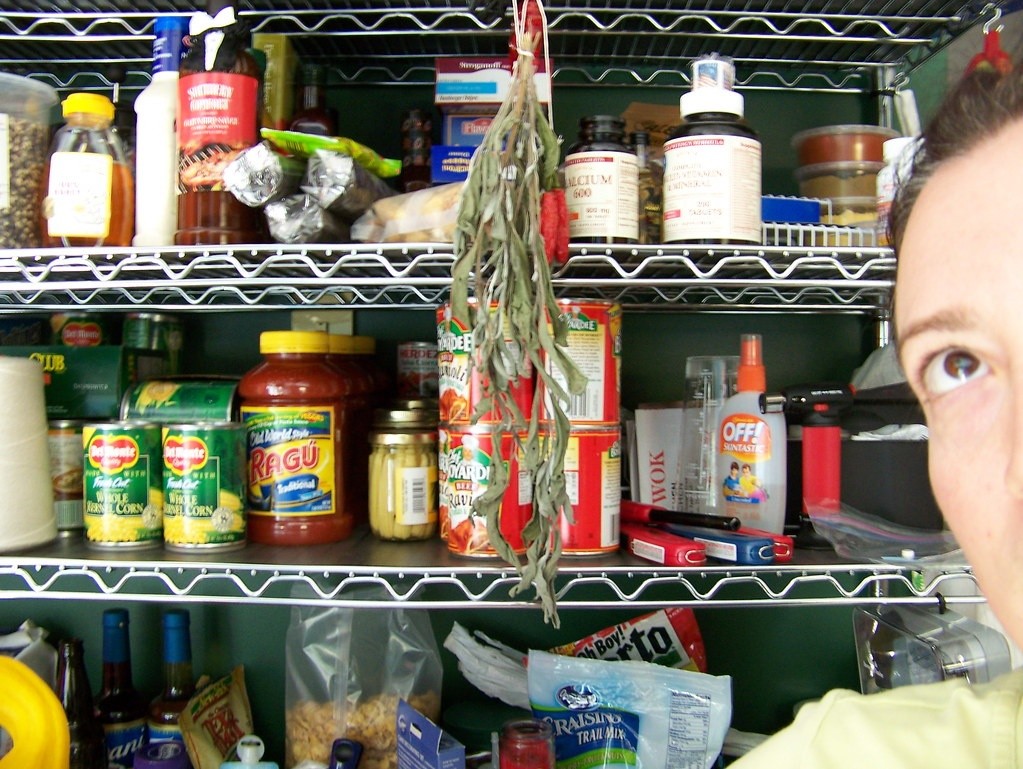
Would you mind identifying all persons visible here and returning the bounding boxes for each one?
[716,63,1023,769]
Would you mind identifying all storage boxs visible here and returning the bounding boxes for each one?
[253,33,299,133]
[431,57,554,184]
[1,343,179,419]
[763,197,821,224]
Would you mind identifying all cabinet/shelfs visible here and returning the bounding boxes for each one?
[0,0,1019,610]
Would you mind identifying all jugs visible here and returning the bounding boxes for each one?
[679,353,739,517]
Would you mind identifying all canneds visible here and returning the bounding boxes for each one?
[30,299,623,564]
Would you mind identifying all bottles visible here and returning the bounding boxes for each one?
[562,53,762,265]
[714,333,789,542]
[0,17,334,249]
[91,608,147,769]
[142,609,198,760]
[56,639,109,769]
